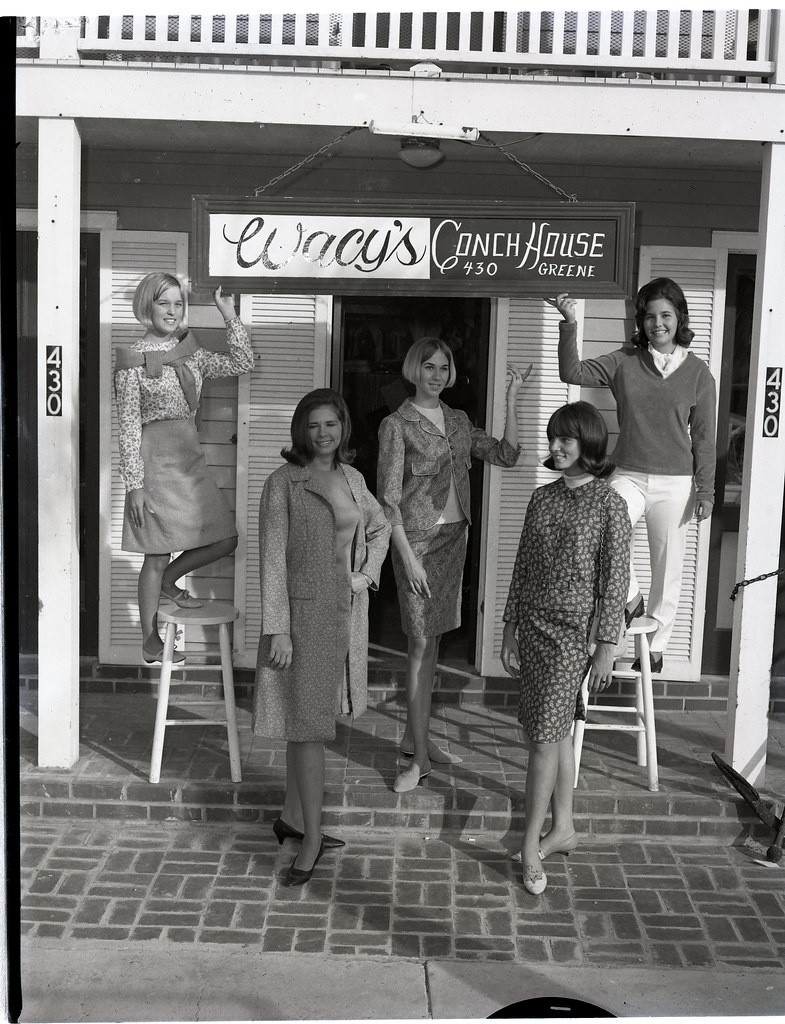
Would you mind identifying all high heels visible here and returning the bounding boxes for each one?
[287,839,325,886]
[273,818,345,848]
[522,865,547,895]
[511,831,577,862]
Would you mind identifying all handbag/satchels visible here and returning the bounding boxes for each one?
[587,596,627,657]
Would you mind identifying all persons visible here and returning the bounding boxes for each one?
[498,401,633,895]
[376,336,532,793]
[544,275,716,673]
[112,272,255,663]
[251,387,393,888]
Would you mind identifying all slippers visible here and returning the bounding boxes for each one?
[400,747,463,764]
[393,770,431,793]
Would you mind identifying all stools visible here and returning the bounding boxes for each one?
[149,602,242,784]
[571,615,659,792]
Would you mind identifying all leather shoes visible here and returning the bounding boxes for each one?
[631,653,662,674]
[624,597,644,630]
[143,644,186,663]
[160,588,204,608]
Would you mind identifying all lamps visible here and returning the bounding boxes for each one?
[400,135,446,170]
